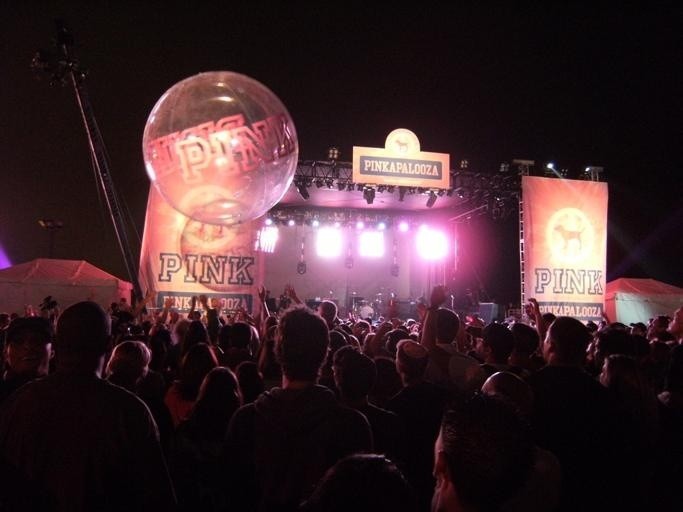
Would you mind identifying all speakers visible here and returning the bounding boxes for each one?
[307,301,321,311]
[265,298,289,316]
[480,303,504,327]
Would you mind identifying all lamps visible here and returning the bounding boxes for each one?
[457,159,469,170]
[327,146,339,161]
[293,175,501,209]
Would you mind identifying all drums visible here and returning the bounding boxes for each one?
[356,298,384,320]
[350,297,364,301]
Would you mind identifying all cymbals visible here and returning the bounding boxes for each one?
[347,291,360,295]
[373,293,386,296]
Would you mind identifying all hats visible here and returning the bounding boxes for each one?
[396,339,432,359]
[630,322,647,330]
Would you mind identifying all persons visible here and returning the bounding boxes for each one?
[0,284,683,512]
